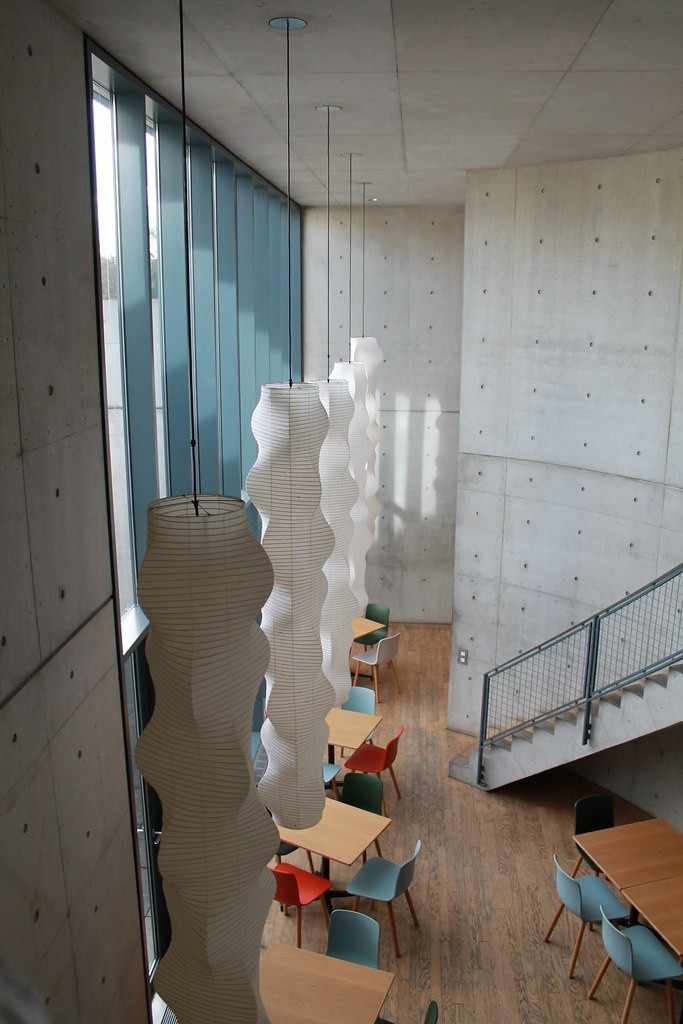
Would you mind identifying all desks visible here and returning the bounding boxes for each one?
[324,707,383,791]
[349,616,386,682]
[271,798,391,920]
[572,818,683,954]
[254,942,397,1024]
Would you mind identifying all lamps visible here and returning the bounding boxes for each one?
[133,0,384,1024]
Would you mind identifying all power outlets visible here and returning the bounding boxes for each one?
[458,647,468,665]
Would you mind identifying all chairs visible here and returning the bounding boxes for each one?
[340,773,391,864]
[345,839,422,958]
[375,1000,438,1024]
[352,632,401,703]
[341,686,383,779]
[543,854,629,978]
[344,725,404,817]
[324,908,380,972]
[354,604,397,674]
[570,793,615,882]
[266,861,334,949]
[588,905,683,1024]
